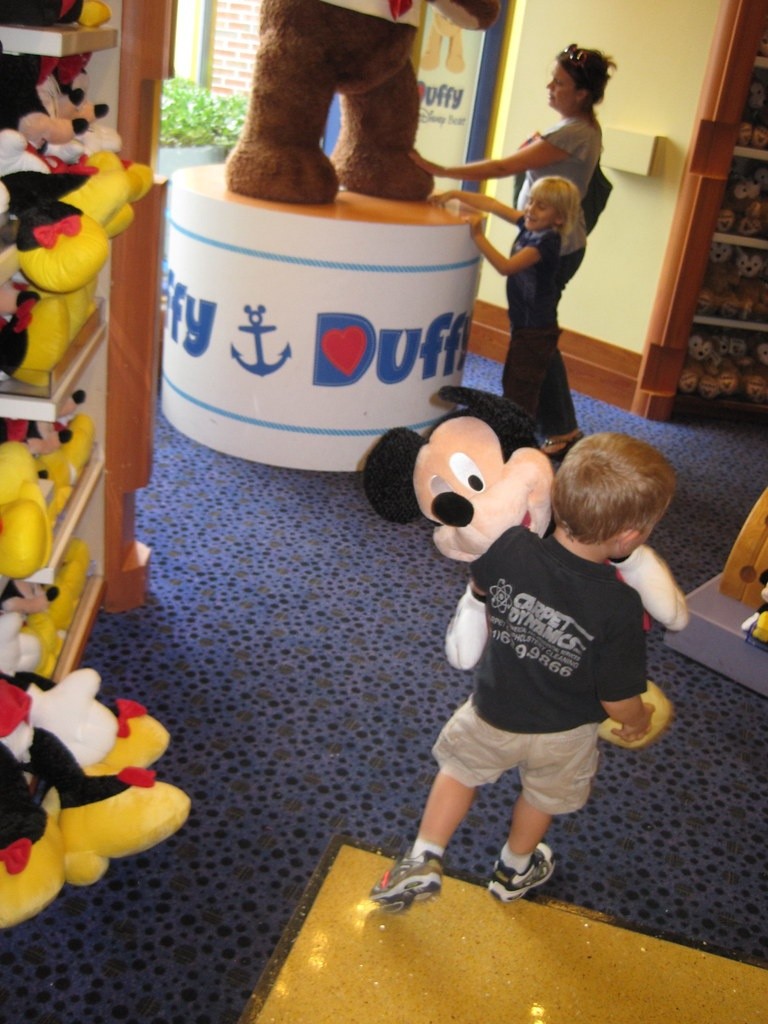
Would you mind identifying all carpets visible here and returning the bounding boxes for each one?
[236,835,768,1024]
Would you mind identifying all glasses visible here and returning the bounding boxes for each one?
[566,44,590,90]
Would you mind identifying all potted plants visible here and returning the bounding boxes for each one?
[157,76,247,174]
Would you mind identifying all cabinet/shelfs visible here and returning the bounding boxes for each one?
[673,54,768,414]
[0,22,108,685]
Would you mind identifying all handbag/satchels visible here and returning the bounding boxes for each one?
[513,147,613,238]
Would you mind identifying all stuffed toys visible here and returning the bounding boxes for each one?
[676,31,768,401]
[0,0,192,933]
[362,386,688,748]
[228,0,501,206]
[742,570,768,641]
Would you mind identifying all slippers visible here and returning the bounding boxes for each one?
[540,430,584,456]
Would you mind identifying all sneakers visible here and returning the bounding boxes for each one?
[489,843,555,903]
[369,844,443,914]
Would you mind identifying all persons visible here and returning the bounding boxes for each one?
[368,433,677,914]
[430,175,584,422]
[407,43,613,456]
